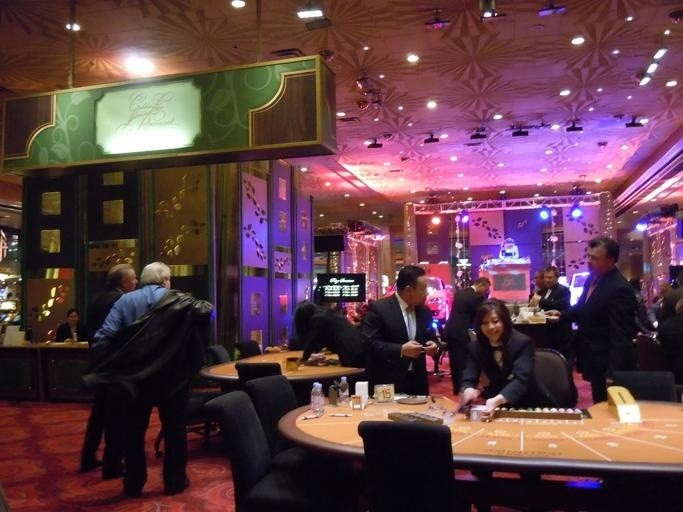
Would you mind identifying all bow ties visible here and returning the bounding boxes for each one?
[492,345,503,351]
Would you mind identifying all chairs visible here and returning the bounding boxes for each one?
[208,344,230,362]
[287,336,306,351]
[248,376,304,463]
[526,350,577,405]
[207,393,309,510]
[155,388,212,459]
[346,422,470,507]
[612,370,677,400]
[431,327,450,376]
[234,340,262,356]
[235,361,276,383]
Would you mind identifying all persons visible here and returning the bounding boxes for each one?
[293,300,366,397]
[81,263,139,480]
[458,297,559,512]
[55,308,91,342]
[445,265,683,395]
[359,265,444,395]
[544,237,640,405]
[91,261,215,497]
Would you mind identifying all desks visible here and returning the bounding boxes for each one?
[511,321,573,348]
[201,345,365,401]
[276,395,683,512]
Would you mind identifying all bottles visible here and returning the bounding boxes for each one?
[312,384,324,414]
[338,377,349,402]
[310,383,318,408]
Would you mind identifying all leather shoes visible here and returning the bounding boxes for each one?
[83,460,125,479]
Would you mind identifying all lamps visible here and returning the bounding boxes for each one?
[539,204,552,221]
[633,32,668,88]
[479,0,497,17]
[571,202,583,219]
[352,222,386,241]
[357,77,381,107]
[367,118,644,148]
[461,211,470,224]
[296,2,326,19]
[431,212,440,225]
